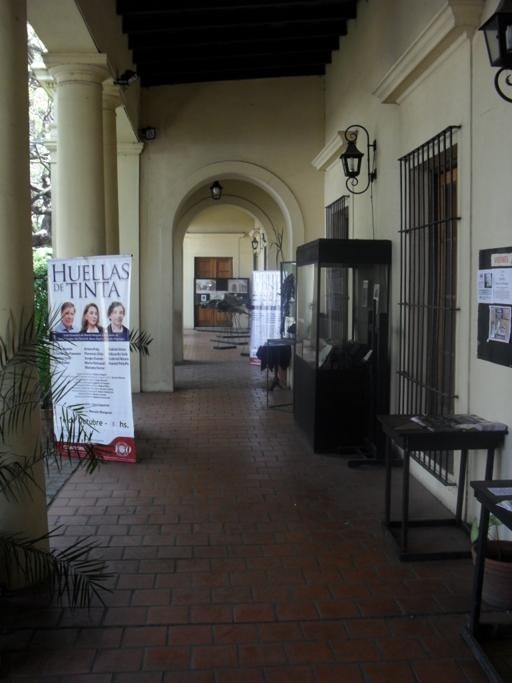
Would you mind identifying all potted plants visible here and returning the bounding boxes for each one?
[470,510,512,609]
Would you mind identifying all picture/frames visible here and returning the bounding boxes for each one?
[194,277,249,307]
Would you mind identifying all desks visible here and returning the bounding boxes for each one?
[378,414,509,562]
[255,344,291,392]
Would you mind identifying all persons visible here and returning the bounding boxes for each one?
[106,301,129,333]
[78,302,104,334]
[54,302,79,333]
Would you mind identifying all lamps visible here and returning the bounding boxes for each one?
[113,70,138,86]
[339,125,376,194]
[138,125,155,141]
[478,1,512,103]
[210,180,224,200]
[252,237,263,250]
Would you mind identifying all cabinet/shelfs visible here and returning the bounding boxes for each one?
[458,480,512,683]
